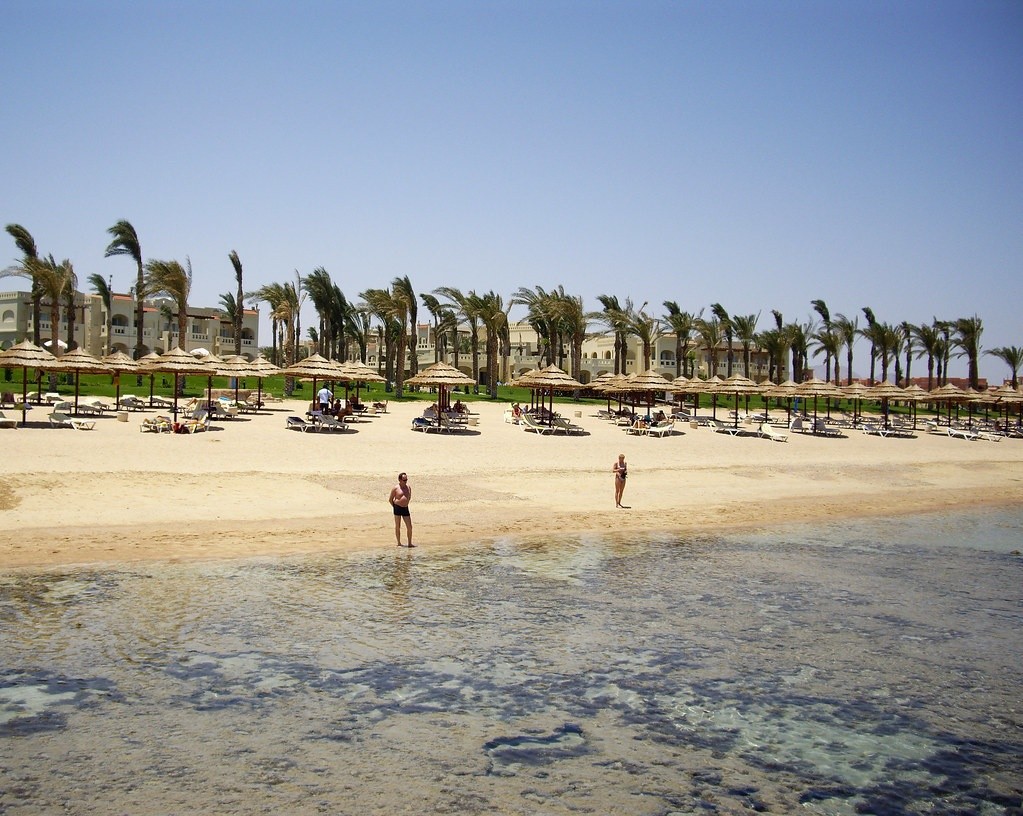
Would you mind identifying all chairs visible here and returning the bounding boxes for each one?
[0,389,264,434]
[285,399,1023,442]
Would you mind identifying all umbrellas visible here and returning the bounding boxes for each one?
[279,352,388,430]
[403,361,477,433]
[758,378,1023,434]
[671,373,767,429]
[585,369,681,426]
[513,363,587,427]
[0,339,283,427]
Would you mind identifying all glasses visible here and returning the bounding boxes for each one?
[401,479,407,482]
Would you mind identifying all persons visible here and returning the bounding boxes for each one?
[389,472,417,548]
[317,385,333,414]
[334,399,341,410]
[658,410,664,421]
[175,418,198,427]
[613,454,628,508]
[338,401,352,419]
[512,403,520,421]
[453,399,463,412]
[350,394,356,403]
[143,416,170,424]
[523,405,528,413]
